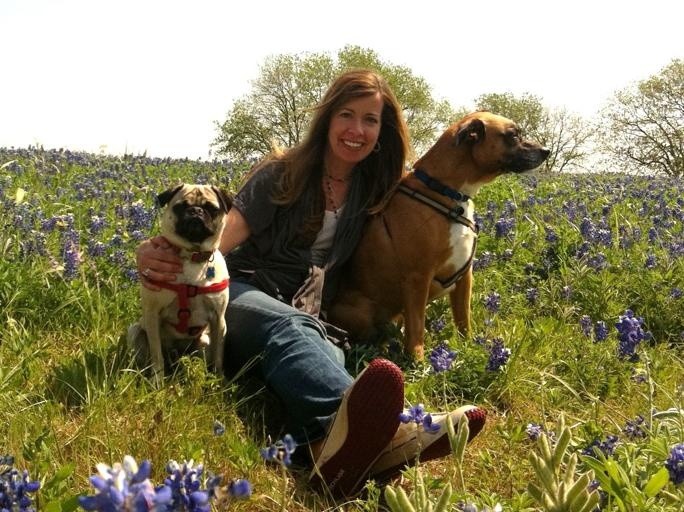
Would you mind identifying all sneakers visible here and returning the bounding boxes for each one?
[306,358,405,501]
[359,405,486,488]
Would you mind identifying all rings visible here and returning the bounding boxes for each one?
[142,268,150,277]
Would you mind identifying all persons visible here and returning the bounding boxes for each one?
[136,69,489,502]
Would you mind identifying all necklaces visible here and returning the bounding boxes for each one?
[325,177,339,220]
[327,172,351,183]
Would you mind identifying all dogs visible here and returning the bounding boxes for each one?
[124,181,235,391]
[325,109,552,370]
